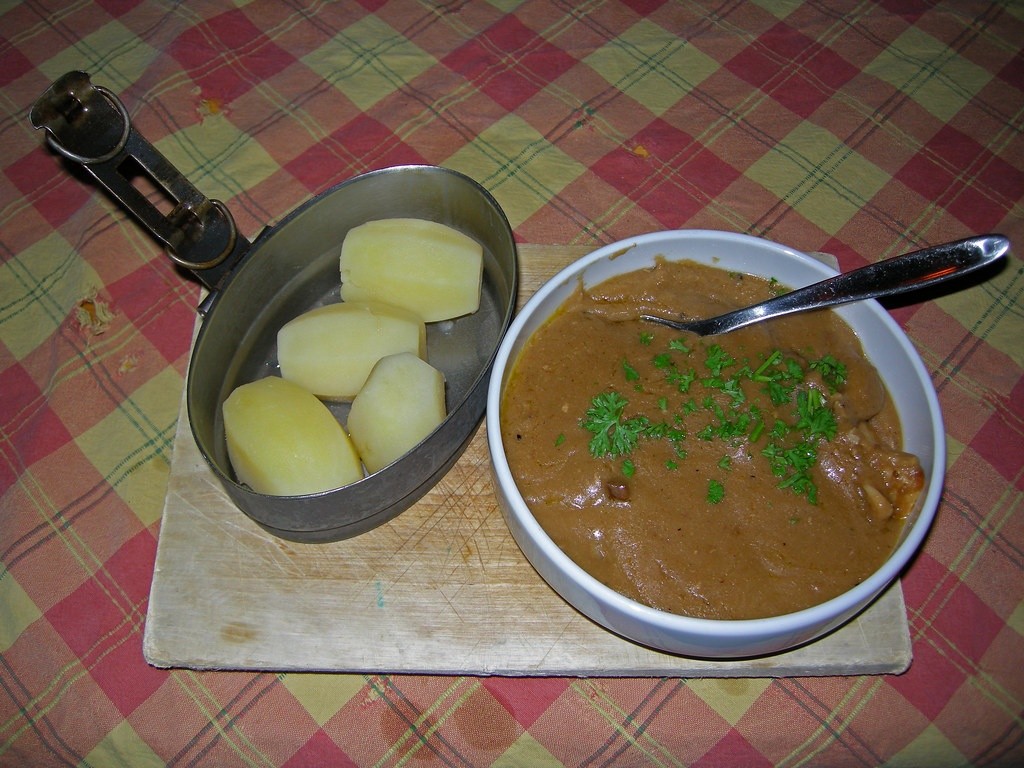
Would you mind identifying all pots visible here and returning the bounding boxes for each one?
[27,69,521,545]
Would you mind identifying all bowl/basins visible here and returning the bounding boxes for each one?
[488,229,946,656]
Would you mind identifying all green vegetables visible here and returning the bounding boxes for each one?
[579,333,848,505]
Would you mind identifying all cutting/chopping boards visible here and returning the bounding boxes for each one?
[142,240,914,676]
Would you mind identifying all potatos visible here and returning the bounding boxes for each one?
[223,217,483,496]
